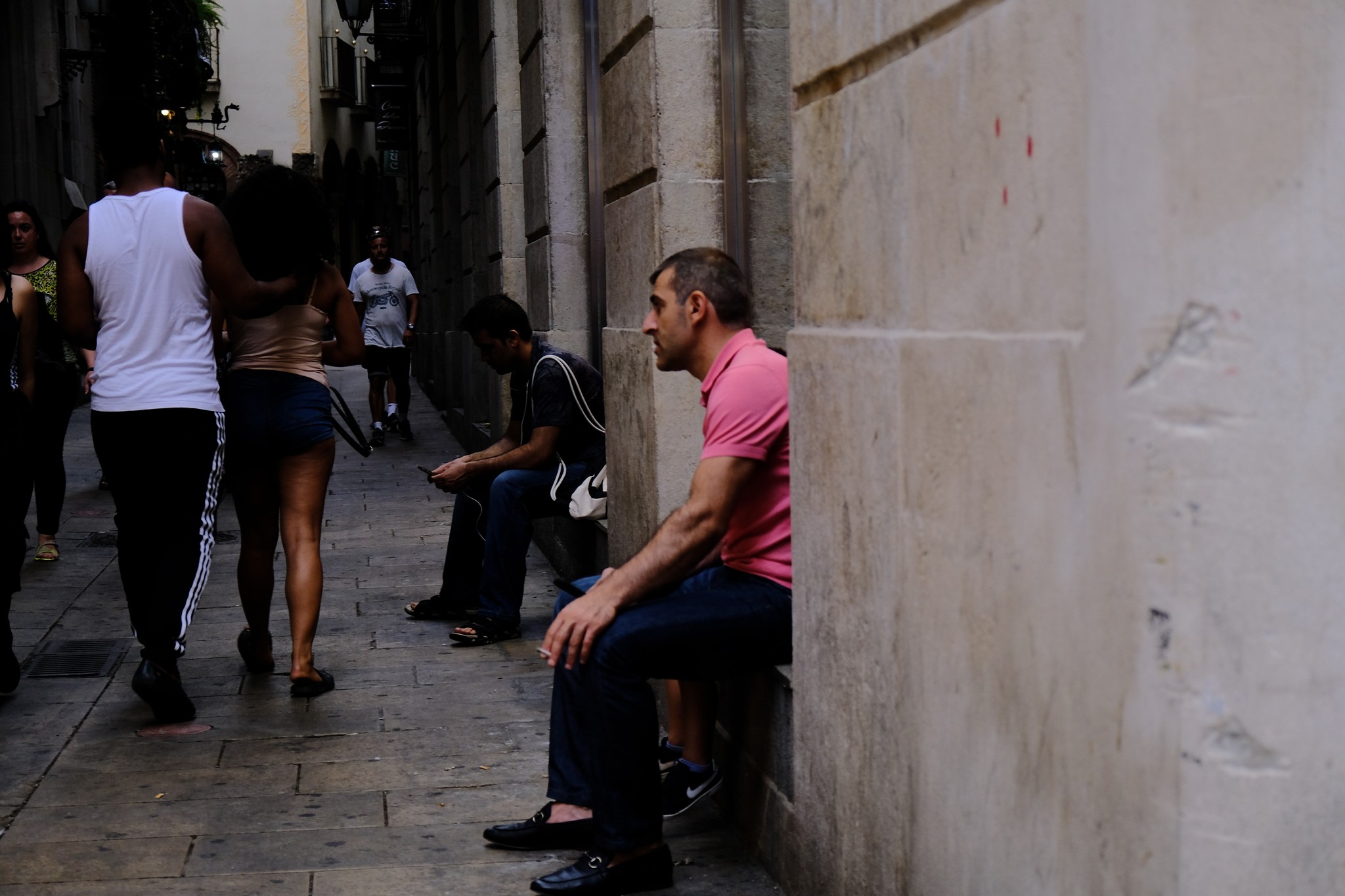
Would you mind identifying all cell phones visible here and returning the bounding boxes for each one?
[418,466,437,476]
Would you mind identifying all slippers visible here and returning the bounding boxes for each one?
[291,668,335,697]
[237,626,276,676]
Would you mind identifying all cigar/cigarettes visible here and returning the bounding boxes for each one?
[535,647,551,658]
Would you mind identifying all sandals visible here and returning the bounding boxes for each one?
[403,593,479,618]
[34,542,60,561]
[449,620,523,643]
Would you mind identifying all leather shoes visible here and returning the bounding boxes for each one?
[529,844,674,895]
[483,800,594,850]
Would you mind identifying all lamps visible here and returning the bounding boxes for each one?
[336,0,424,44]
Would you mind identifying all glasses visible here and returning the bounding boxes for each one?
[369,230,386,236]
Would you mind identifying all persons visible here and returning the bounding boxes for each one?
[653,677,721,822]
[403,294,605,644]
[482,246,787,896]
[0,97,420,722]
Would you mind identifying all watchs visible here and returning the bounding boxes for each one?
[406,324,415,331]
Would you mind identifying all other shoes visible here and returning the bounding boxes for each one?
[100,477,109,490]
[369,428,385,446]
[386,413,398,424]
[659,737,684,773]
[132,660,196,723]
[397,419,415,440]
[660,757,722,817]
